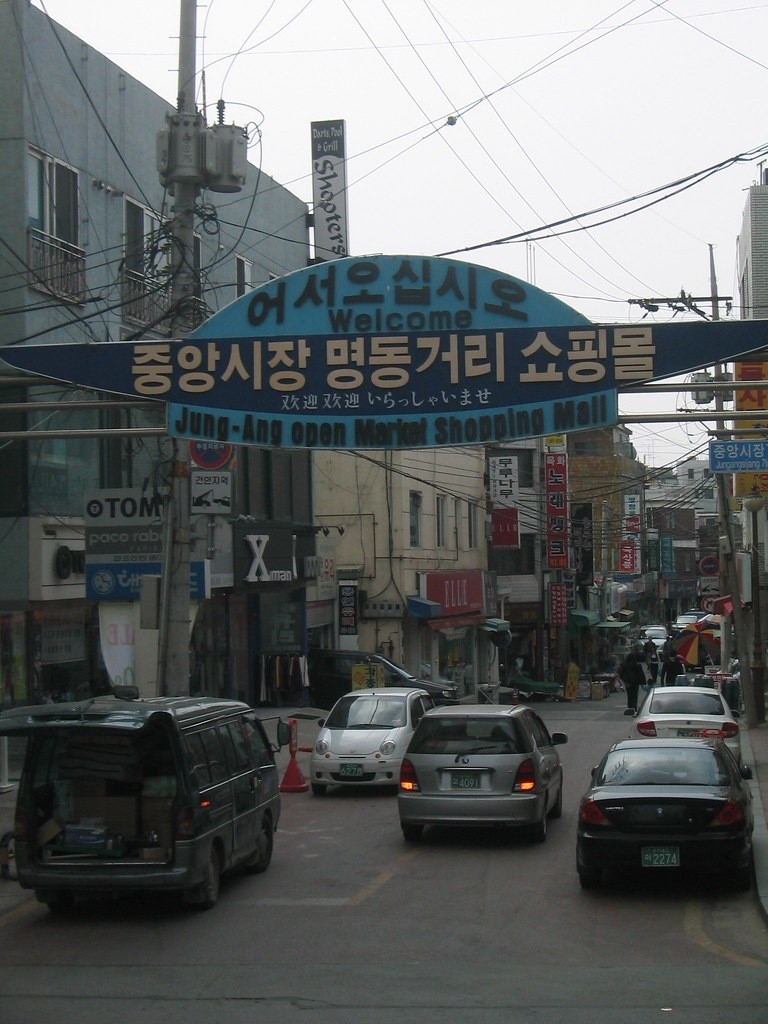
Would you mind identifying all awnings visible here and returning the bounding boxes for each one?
[567,608,635,633]
[480,618,510,632]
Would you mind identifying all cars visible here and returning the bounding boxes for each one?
[629,625,673,662]
[398,704,570,842]
[668,611,706,633]
[575,737,754,889]
[310,685,437,796]
[623,684,741,764]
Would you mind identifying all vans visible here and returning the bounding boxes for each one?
[0,684,292,916]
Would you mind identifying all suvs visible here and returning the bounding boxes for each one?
[292,649,460,708]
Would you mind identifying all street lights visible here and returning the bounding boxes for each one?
[743,482,765,720]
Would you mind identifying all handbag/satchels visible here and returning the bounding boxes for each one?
[659,665,662,676]
[636,664,646,685]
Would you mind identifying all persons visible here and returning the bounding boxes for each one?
[597,635,686,713]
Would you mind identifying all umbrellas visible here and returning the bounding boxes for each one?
[672,620,722,666]
